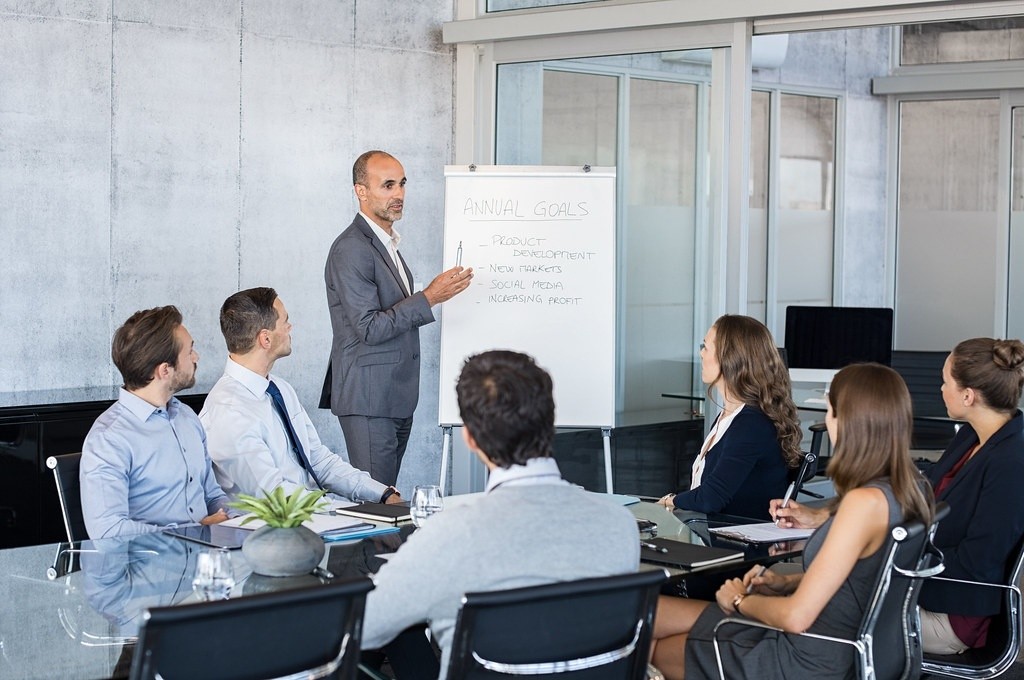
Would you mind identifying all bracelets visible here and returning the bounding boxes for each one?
[663,492,676,505]
[731,593,752,608]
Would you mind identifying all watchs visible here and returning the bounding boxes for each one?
[380,485,401,505]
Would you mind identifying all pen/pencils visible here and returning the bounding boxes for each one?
[640,541,669,553]
[747,566,766,594]
[798,489,826,498]
[456,241,463,276]
[317,566,334,578]
[774,481,795,526]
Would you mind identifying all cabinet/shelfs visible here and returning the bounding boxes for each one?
[0,383,214,550]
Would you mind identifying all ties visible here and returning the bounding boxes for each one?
[266,380,327,496]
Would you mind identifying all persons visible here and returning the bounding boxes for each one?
[765,338,1024,657]
[80,304,246,543]
[362,350,641,679]
[317,150,475,488]
[81,534,207,642]
[195,287,408,516]
[649,310,807,603]
[646,362,935,680]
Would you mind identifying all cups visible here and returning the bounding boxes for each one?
[410,485,443,529]
[191,548,235,602]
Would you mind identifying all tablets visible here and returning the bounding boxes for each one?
[161,524,257,550]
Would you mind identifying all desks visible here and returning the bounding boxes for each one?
[662,382,972,425]
[1,492,813,680]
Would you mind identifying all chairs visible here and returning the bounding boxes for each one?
[786,450,817,501]
[489,417,705,496]
[46,453,160,648]
[920,545,1024,680]
[891,351,968,450]
[358,568,670,680]
[47,543,80,580]
[713,501,952,680]
[131,576,375,680]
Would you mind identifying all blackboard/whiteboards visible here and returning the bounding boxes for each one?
[438,164,618,428]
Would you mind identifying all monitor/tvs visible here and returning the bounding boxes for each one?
[784,305,894,404]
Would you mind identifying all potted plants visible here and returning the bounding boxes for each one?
[222,485,331,576]
